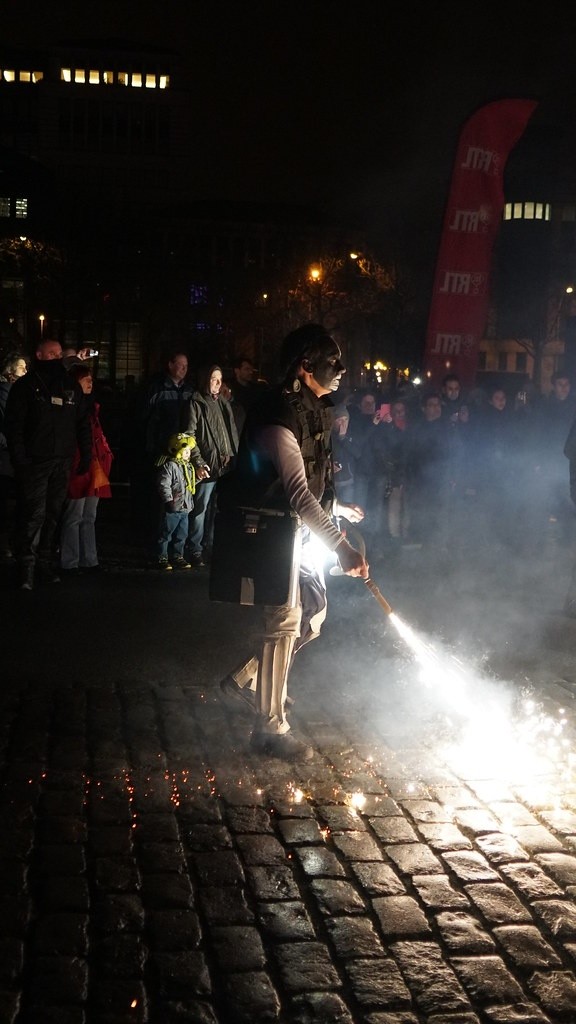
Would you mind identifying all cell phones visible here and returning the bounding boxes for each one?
[379,403,391,420]
[518,392,526,404]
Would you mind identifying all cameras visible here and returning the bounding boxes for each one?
[88,350,99,357]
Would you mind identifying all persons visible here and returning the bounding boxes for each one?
[136,354,576,575]
[0,338,116,592]
[214,320,372,762]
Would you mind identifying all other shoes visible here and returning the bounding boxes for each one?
[220,673,259,716]
[248,728,312,761]
[66,565,109,579]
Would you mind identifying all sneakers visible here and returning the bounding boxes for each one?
[189,553,206,567]
[172,556,192,569]
[155,555,172,570]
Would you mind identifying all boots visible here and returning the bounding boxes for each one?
[37,558,61,586]
[15,555,37,592]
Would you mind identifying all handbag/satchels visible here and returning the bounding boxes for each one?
[210,497,298,609]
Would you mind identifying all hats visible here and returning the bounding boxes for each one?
[166,432,196,458]
